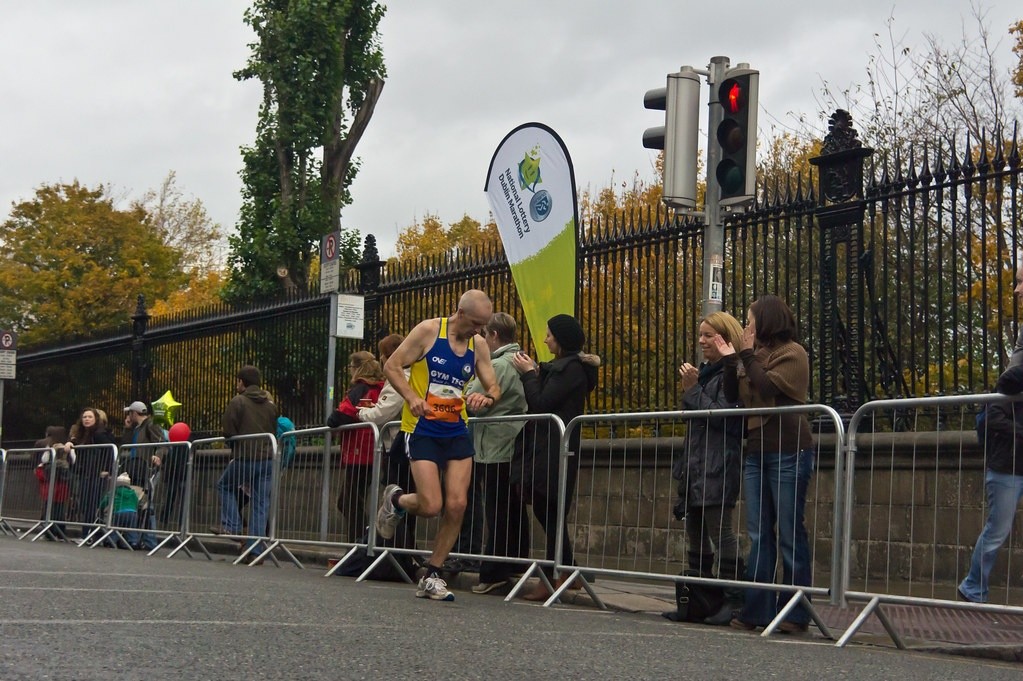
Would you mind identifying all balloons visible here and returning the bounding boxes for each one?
[151,390,182,427]
[169,422,190,442]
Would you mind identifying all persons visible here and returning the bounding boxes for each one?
[715,296,811,634]
[672,310,743,624]
[957,264,1023,603]
[328,312,600,602]
[99,472,144,550]
[32,401,188,550]
[209,366,295,565]
[375,290,500,600]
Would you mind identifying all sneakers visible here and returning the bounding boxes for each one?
[377,484,405,534]
[416,576,454,600]
[472,579,507,593]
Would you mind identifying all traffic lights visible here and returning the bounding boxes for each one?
[714,64,757,206]
[641,74,700,211]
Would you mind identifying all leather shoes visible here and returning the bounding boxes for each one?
[234,553,264,565]
[209,527,242,542]
[559,572,583,590]
[705,598,739,626]
[776,621,808,633]
[524,579,566,601]
[731,618,757,631]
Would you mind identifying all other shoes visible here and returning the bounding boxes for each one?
[40,534,51,540]
[57,534,65,541]
[71,538,156,551]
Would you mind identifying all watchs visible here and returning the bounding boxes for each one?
[484,394,495,405]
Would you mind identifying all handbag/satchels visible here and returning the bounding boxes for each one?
[675,569,723,620]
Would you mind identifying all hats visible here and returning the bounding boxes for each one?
[235,366,260,384]
[124,401,147,413]
[547,314,585,349]
[116,472,130,483]
